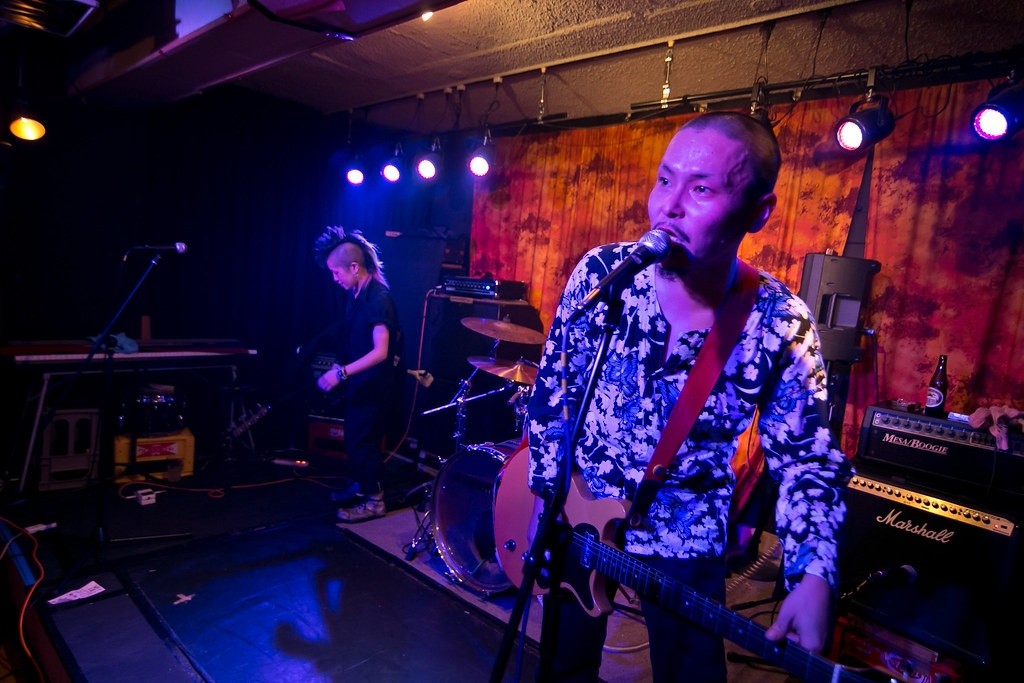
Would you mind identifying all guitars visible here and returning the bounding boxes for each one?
[493,442,903,683]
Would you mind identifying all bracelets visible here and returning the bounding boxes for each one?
[336,363,348,381]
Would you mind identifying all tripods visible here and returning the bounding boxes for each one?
[44,250,195,598]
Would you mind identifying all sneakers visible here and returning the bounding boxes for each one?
[336,491,386,523]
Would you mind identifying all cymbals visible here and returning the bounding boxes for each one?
[459,316,547,345]
[466,355,539,385]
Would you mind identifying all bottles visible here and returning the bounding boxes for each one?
[924,354,948,419]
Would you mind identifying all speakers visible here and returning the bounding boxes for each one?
[800,253,881,359]
[304,353,346,423]
[834,460,1024,683]
[413,293,542,459]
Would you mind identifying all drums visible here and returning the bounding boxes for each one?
[430,443,517,595]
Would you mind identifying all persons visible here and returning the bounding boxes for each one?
[523,111,850,683]
[318,235,398,524]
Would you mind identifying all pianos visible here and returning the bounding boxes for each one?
[0,338,249,494]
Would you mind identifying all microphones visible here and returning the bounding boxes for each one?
[567,230,672,326]
[869,565,917,585]
[128,242,189,254]
